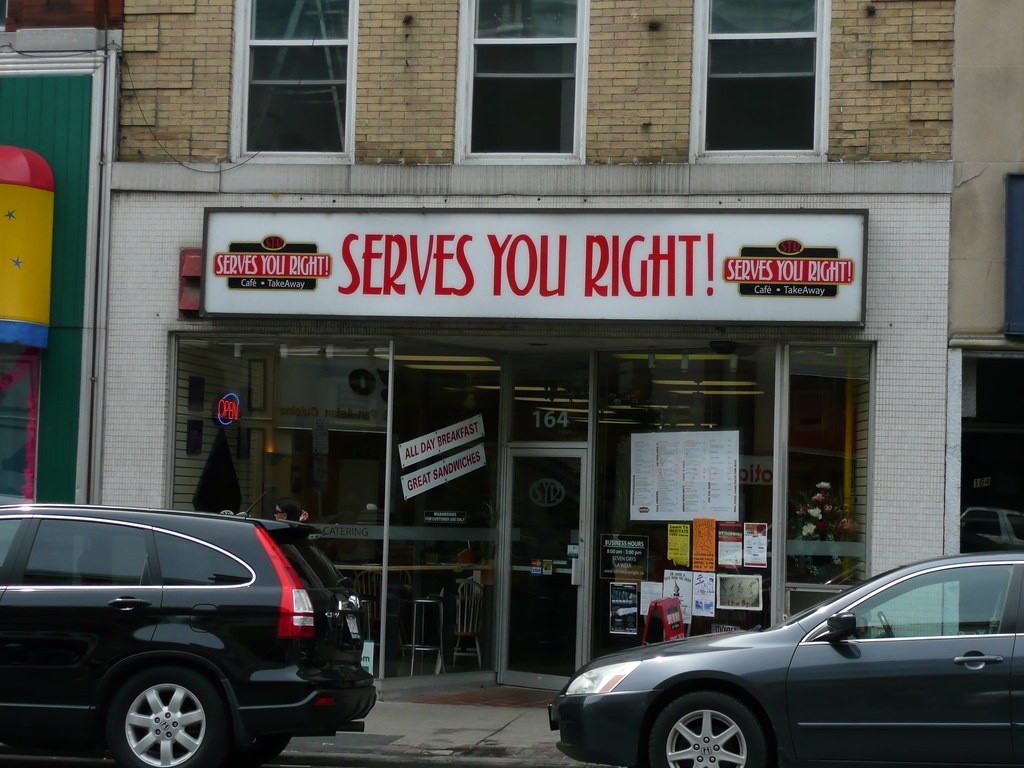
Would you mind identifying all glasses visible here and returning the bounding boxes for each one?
[275,509,285,514]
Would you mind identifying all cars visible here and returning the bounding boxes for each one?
[960,506,1024,546]
[545,554,1024,768]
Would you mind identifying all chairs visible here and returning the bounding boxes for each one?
[350,571,483,670]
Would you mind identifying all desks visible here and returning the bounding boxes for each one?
[331,563,494,674]
[400,597,447,676]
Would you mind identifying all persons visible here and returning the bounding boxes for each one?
[274,496,308,521]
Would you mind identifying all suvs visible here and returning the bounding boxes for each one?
[0,501,377,768]
